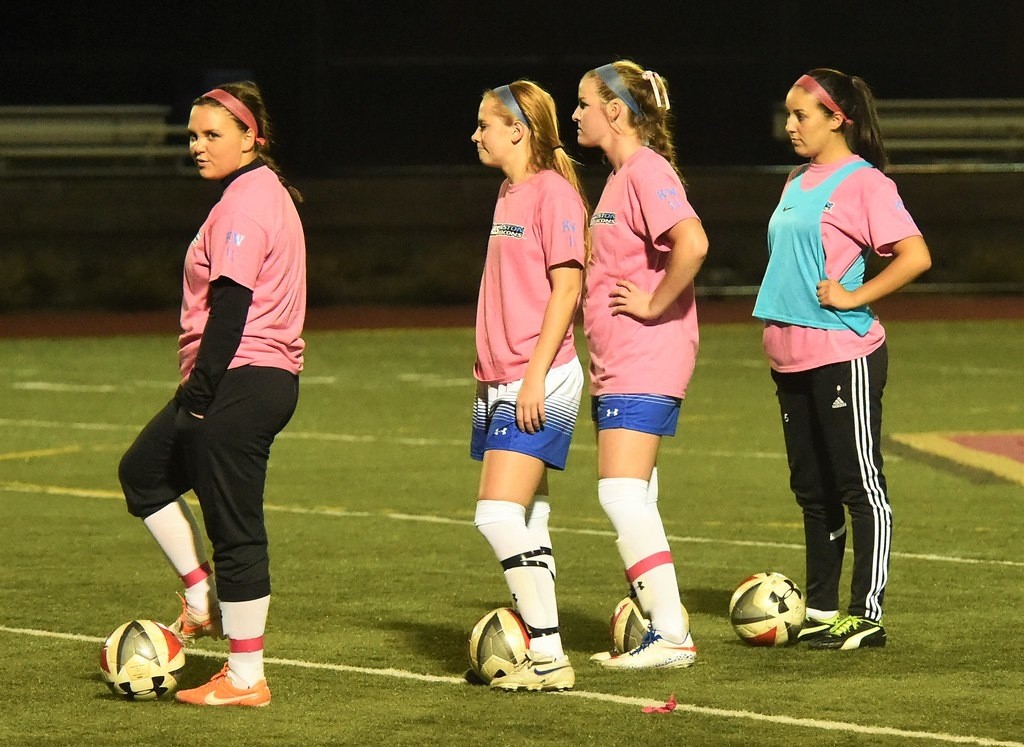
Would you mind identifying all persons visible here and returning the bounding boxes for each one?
[471,81,590,692]
[572,59,709,672]
[116,81,308,707]
[751,69,931,651]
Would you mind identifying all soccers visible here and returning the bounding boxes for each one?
[467,606,530,685]
[98,619,187,702]
[728,571,806,648]
[609,594,689,656]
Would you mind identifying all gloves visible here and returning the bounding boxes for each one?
[157,406,205,460]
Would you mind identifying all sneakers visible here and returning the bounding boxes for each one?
[805,613,887,650]
[168,601,228,644]
[490,649,576,692]
[174,661,272,707]
[591,628,697,671]
[783,612,841,648]
